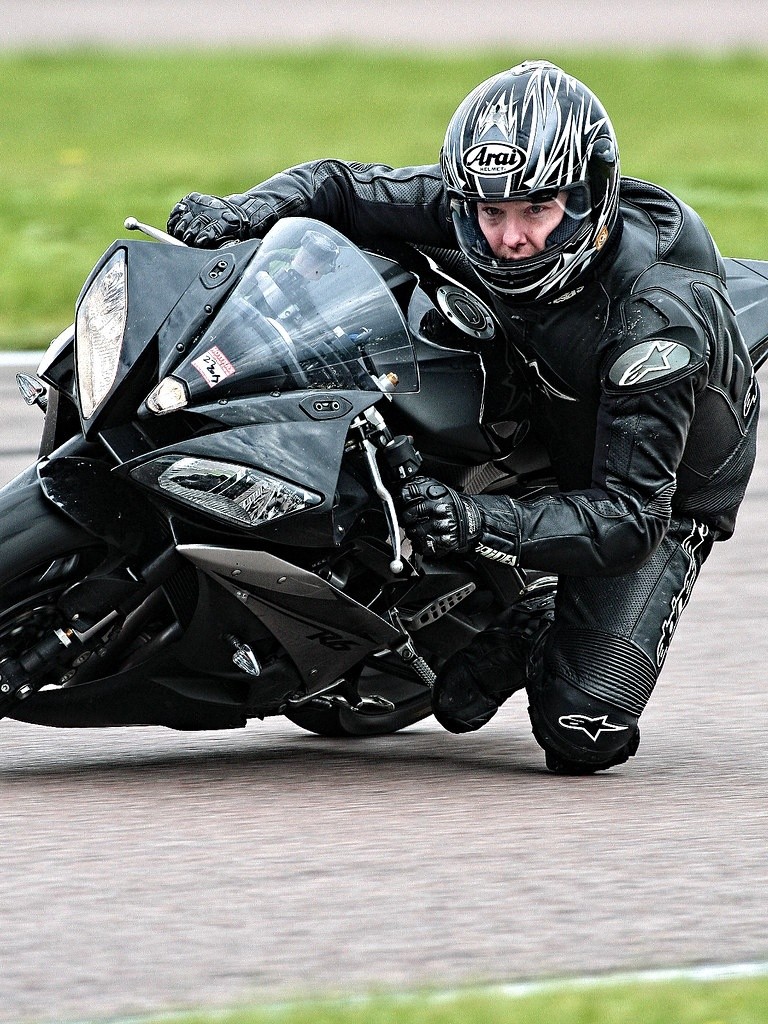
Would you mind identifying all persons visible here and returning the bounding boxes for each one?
[167,59,761,779]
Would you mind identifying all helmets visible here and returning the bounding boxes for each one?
[440,60,621,310]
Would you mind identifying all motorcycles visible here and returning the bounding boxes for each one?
[1,214,767,740]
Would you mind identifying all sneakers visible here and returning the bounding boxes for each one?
[432,577,556,735]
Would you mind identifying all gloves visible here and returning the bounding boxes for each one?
[167,192,279,249]
[393,475,521,570]
